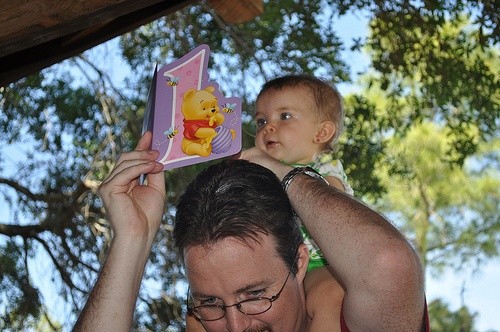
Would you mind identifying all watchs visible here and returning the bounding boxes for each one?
[281,166,330,192]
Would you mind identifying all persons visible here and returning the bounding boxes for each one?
[73,130,429,332]
[255,74,354,332]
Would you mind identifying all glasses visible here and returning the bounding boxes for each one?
[186,255,296,321]
